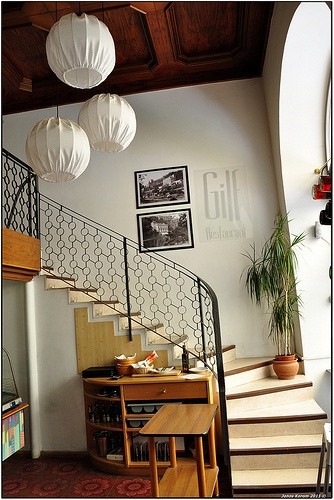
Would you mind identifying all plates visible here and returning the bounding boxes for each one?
[150,367,174,373]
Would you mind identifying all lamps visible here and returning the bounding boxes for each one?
[25,1,137,182]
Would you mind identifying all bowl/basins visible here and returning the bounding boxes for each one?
[135,367,149,374]
[117,364,133,377]
[116,358,136,365]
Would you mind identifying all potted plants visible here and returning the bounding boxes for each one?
[239,209,308,380]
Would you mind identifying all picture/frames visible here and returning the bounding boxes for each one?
[136,208,194,253]
[134,166,191,209]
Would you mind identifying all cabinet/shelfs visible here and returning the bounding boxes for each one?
[82,369,220,497]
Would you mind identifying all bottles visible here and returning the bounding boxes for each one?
[182,345,189,373]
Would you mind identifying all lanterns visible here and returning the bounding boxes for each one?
[26,117,90,184]
[46,13,116,89]
[78,93,136,153]
